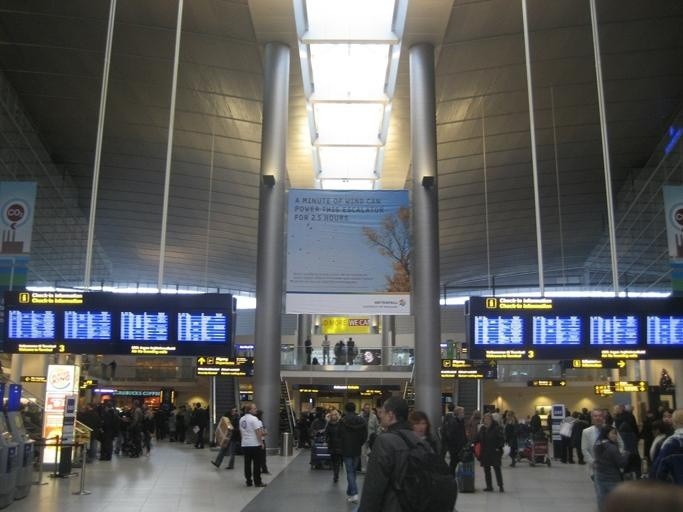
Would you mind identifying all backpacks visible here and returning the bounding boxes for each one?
[392,430,457,512]
[456,471,475,492]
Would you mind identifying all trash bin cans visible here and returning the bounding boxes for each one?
[282,432,293,456]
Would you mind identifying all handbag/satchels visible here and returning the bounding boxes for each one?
[501,441,512,467]
[472,440,481,460]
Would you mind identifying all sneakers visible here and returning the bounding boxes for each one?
[578,460,586,465]
[569,461,575,464]
[347,494,358,503]
[499,487,504,492]
[483,488,493,492]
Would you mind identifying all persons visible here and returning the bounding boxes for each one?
[407,411,441,456]
[440,404,520,492]
[560,403,683,512]
[77,386,208,464]
[298,401,380,503]
[210,399,272,488]
[333,337,354,365]
[321,334,330,364]
[304,335,312,364]
[359,396,436,512]
[529,410,553,443]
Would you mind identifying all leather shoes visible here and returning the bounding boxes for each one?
[255,482,267,487]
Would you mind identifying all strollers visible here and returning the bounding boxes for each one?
[515,432,552,469]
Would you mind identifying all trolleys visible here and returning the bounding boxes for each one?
[310,428,334,470]
